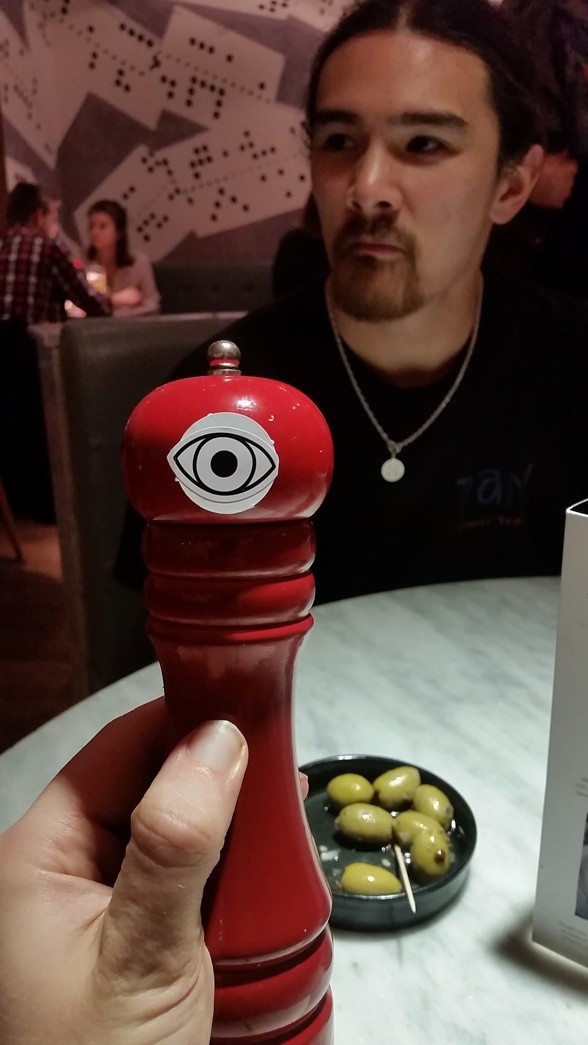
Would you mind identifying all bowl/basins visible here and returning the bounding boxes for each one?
[298,751,477,933]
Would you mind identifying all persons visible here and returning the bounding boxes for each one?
[74,197,161,318]
[116,0,588,680]
[0,694,249,1045]
[0,179,100,528]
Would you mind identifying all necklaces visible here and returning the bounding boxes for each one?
[325,271,484,482]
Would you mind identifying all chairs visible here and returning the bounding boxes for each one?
[60,313,242,699]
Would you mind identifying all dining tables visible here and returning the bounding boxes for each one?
[0,576,588,1045]
